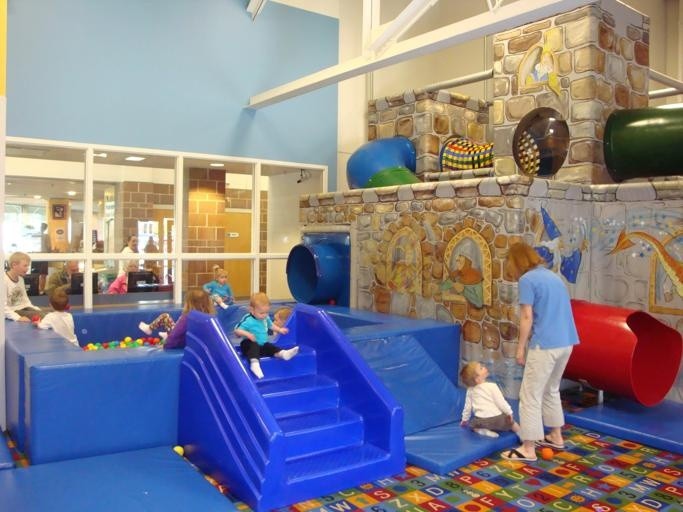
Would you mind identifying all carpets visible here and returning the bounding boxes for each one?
[163,411,683,511]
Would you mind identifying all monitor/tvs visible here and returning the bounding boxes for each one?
[71,273,99,295]
[128,272,153,292]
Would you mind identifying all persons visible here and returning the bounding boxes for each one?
[112,234,141,279]
[457,360,520,439]
[29,223,58,295]
[31,288,80,350]
[54,259,79,294]
[272,308,294,337]
[105,259,141,297]
[202,263,238,311]
[498,240,580,460]
[0,250,48,324]
[232,290,298,380]
[137,288,213,352]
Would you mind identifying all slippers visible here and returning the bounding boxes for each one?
[535,434,565,449]
[500,447,537,462]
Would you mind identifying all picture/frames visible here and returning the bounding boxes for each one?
[51,203,66,221]
